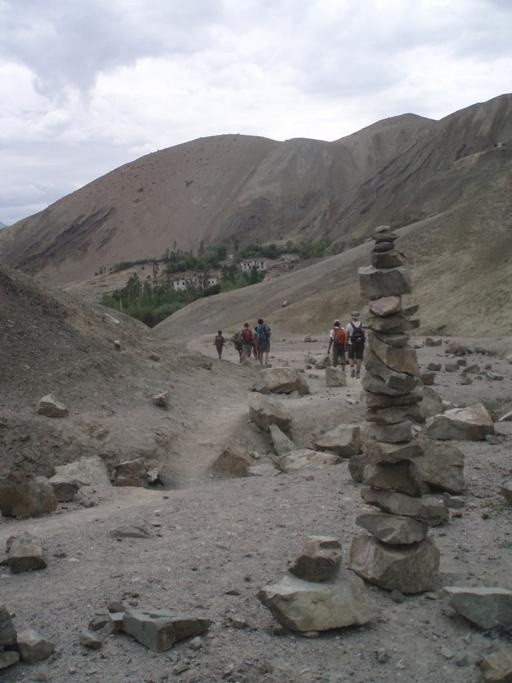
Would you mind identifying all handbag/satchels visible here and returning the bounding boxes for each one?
[239,330,246,344]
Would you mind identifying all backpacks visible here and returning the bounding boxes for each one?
[333,328,346,345]
[259,326,268,343]
[350,322,365,350]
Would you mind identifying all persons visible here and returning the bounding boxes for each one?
[345,310,366,378]
[237,321,257,364]
[253,326,260,361]
[214,328,225,359]
[230,329,242,361]
[327,319,347,371]
[256,318,271,367]
